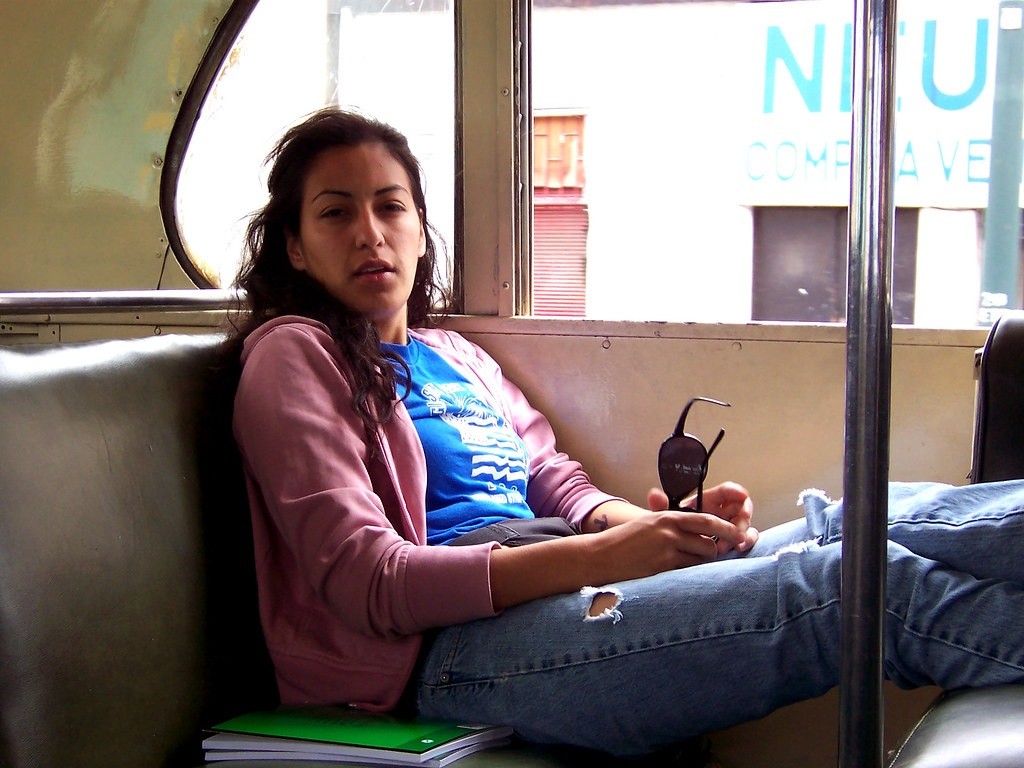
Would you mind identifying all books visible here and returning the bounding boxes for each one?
[202,708,515,768]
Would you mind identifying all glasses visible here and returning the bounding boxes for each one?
[657,397,733,541]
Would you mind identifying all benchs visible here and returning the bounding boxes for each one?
[884,315,1024,768]
[0,329,720,768]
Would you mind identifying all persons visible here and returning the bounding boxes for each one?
[219,102,1023,757]
